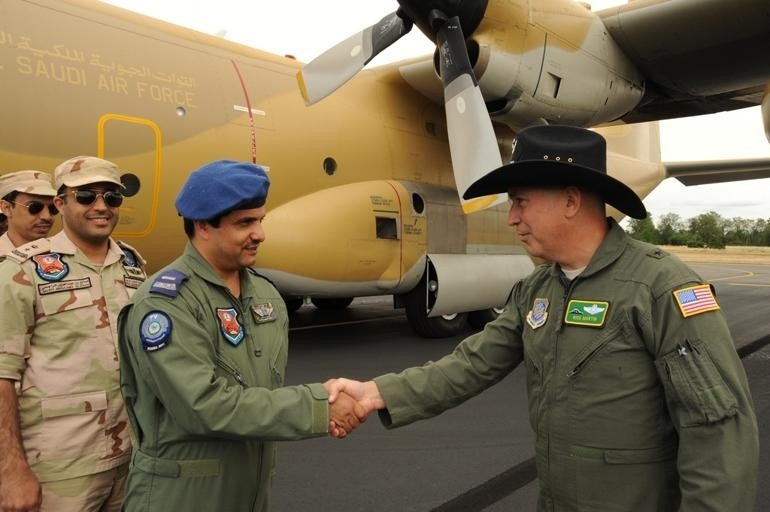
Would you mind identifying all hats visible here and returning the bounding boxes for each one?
[463,125,646,219]
[54,155,126,197]
[0,171,58,201]
[175,159,270,221]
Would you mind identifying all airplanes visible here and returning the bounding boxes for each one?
[0,0,770,338]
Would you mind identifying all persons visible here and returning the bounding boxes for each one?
[0,155,150,511]
[325,124,759,512]
[117,159,368,512]
[0,170,60,262]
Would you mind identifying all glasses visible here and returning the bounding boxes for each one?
[59,189,123,207]
[11,200,59,215]
